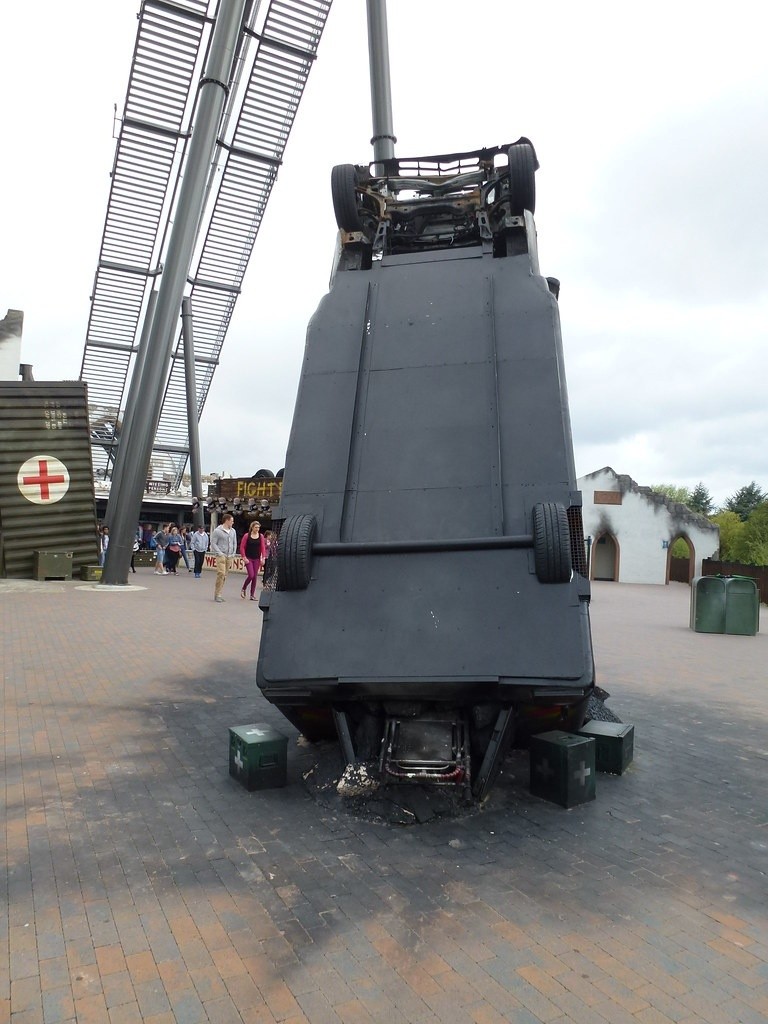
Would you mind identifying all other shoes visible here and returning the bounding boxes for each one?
[162,571,168,574]
[153,571,161,575]
[214,598,222,602]
[133,571,136,573]
[188,570,192,572]
[241,589,246,598]
[221,598,226,602]
[250,595,258,601]
[195,573,200,578]
[174,572,179,576]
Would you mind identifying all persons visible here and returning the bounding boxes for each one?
[239,521,266,601]
[263,530,278,588]
[210,513,237,602]
[98,521,196,576]
[190,524,209,578]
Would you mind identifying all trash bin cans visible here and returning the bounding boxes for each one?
[689,574,761,636]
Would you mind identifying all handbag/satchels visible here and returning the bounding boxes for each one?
[170,545,179,552]
[178,552,183,558]
